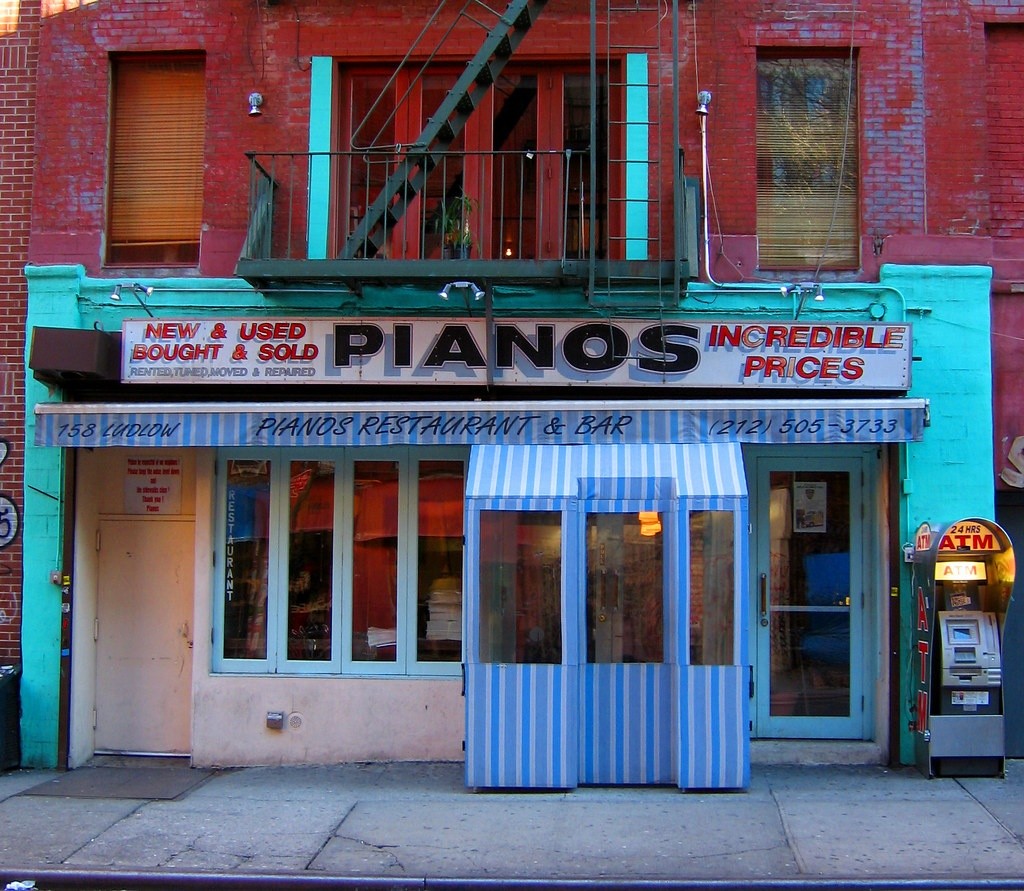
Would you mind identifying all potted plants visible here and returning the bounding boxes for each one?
[435,193,482,259]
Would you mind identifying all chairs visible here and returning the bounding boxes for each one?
[796,552,849,716]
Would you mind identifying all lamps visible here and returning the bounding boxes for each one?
[782,282,823,301]
[439,281,485,300]
[110,282,155,300]
